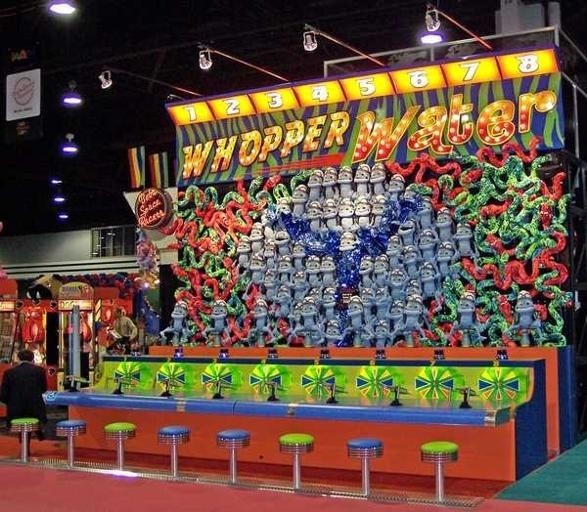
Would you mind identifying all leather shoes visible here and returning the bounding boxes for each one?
[38,436,46,442]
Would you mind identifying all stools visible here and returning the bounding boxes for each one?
[419,440,461,508]
[105,422,137,477]
[217,427,252,488]
[275,433,317,495]
[157,425,192,482]
[345,437,385,501]
[9,417,40,467]
[54,418,86,472]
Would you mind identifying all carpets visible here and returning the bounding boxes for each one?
[493,438,587,508]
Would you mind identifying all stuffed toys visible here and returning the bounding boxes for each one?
[354,136,573,348]
[159,166,372,348]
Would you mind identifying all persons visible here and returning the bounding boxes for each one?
[0,350,48,458]
[113,306,138,355]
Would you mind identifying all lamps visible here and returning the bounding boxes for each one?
[47,0,78,18]
[420,2,493,50]
[98,62,207,105]
[197,40,288,82]
[56,64,86,109]
[302,22,386,66]
[59,130,78,154]
